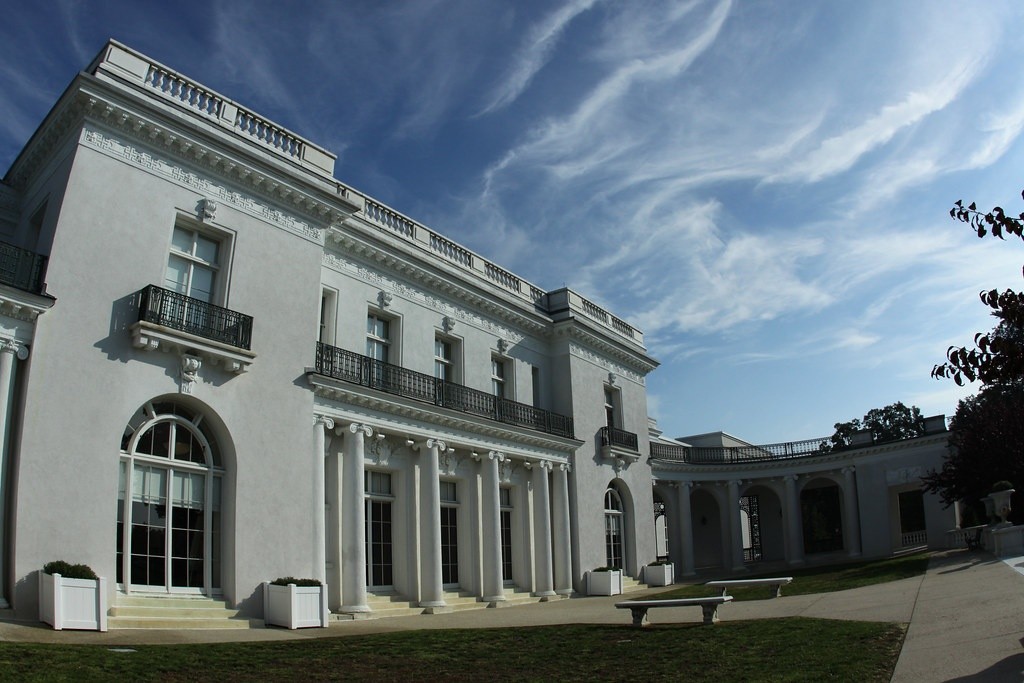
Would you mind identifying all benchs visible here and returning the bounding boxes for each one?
[705,577,793,598]
[614,596,736,624]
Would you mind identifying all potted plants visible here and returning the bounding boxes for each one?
[977,473,1015,529]
[586,567,623,597]
[262,577,329,629]
[38,560,108,632]
[644,561,675,586]
